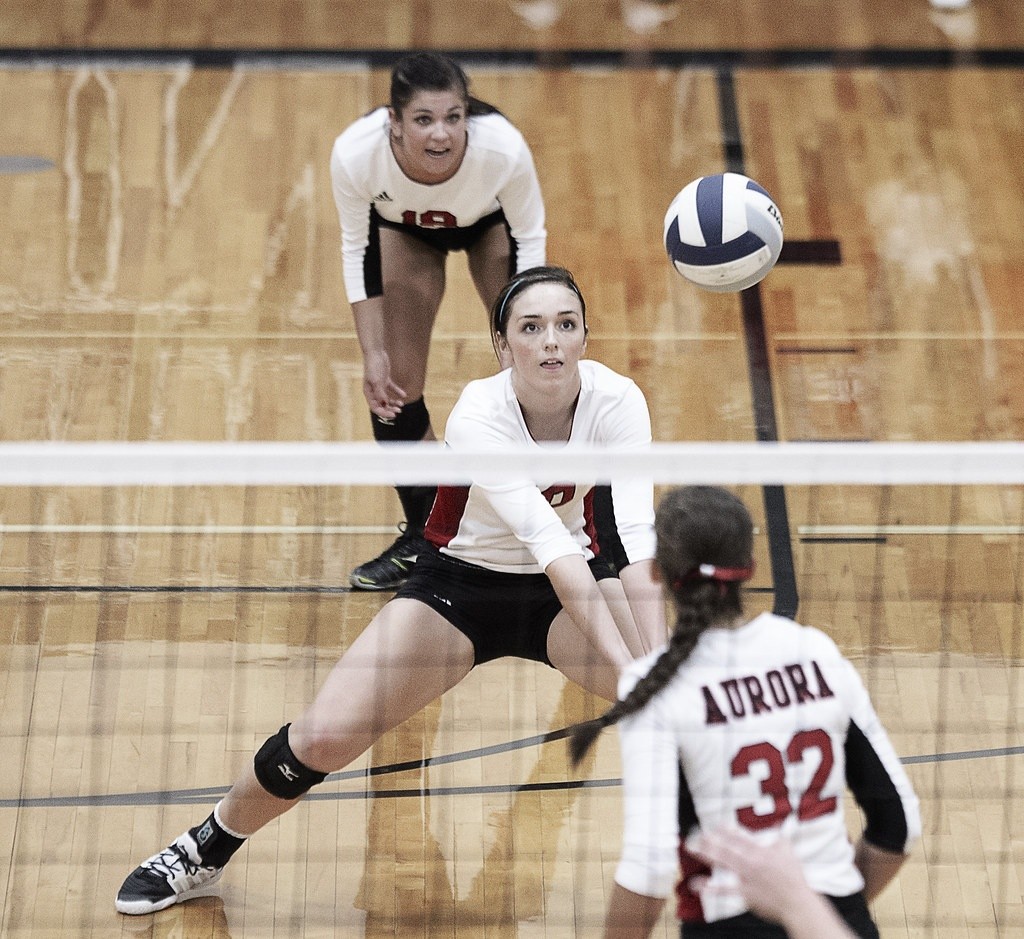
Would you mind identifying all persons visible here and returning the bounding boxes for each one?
[115,267,670,914]
[603,485,923,939]
[329,52,548,596]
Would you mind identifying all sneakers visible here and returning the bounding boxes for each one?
[350,522,426,589]
[116,827,225,916]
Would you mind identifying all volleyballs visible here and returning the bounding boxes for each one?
[663,172,784,294]
